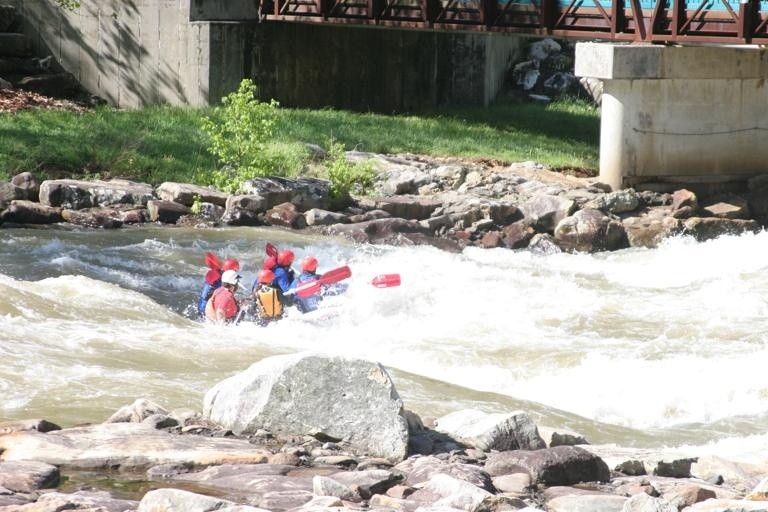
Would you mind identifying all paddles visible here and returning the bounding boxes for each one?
[283,266,352,296]
[205,253,250,293]
[369,274,401,288]
[266,243,300,276]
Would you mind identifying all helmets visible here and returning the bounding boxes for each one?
[221,269,243,285]
[224,259,240,272]
[264,257,277,269]
[302,256,319,273]
[258,269,276,284]
[276,249,295,267]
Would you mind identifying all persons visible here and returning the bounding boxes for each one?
[196,249,349,328]
